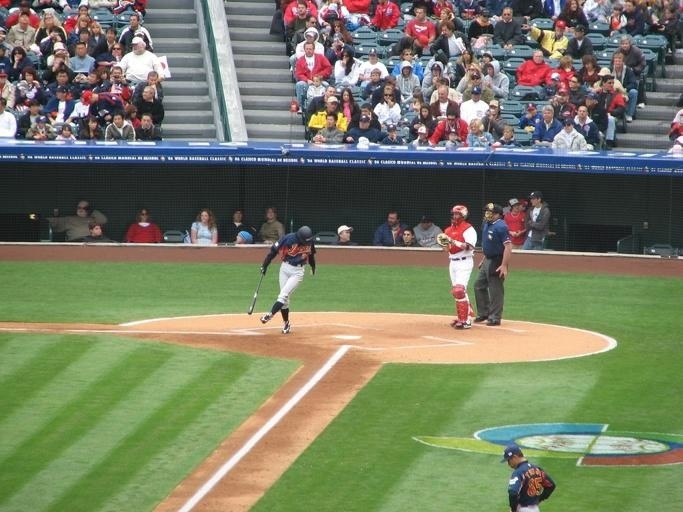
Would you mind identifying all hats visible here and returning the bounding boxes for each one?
[470,72,500,108]
[130,36,142,46]
[555,19,585,33]
[480,8,493,18]
[359,114,458,136]
[585,92,598,100]
[509,191,543,207]
[337,224,354,234]
[500,445,521,463]
[368,47,379,56]
[526,73,574,125]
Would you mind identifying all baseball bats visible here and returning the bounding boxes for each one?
[248,272,265,315]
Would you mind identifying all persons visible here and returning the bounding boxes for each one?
[259,226,316,334]
[501,444,556,512]
[474,203,511,325]
[437,205,478,329]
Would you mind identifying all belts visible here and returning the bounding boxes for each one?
[282,260,302,268]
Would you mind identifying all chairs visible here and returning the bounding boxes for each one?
[1,3,142,41]
[345,0,670,150]
[162,229,185,242]
[312,232,339,245]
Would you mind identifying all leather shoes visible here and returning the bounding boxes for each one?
[485,318,501,326]
[472,316,489,323]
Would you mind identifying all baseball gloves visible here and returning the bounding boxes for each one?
[437,234,453,249]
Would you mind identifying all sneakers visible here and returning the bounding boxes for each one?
[281,322,291,334]
[451,319,471,329]
[260,314,272,324]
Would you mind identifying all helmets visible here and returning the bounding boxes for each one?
[452,205,469,222]
[297,226,313,242]
